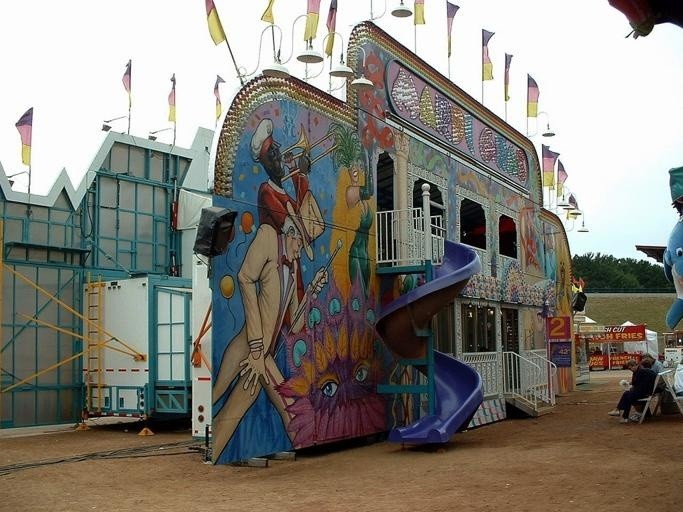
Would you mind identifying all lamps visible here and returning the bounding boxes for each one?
[238,0,413,94]
[539,186,590,235]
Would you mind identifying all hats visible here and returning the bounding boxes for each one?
[284,188,326,262]
[246,117,282,162]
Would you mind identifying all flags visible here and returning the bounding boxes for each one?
[302,0,322,43]
[570,274,586,292]
[14,107,32,165]
[480,27,497,81]
[542,143,582,221]
[502,52,515,102]
[167,76,177,123]
[323,0,338,59]
[445,0,461,59]
[526,72,541,118]
[122,60,134,107]
[203,0,227,45]
[413,0,426,26]
[212,74,226,119]
[260,0,276,24]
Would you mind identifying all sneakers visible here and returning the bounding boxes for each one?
[606,406,643,424]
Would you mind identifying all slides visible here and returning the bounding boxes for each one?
[375,238,483,444]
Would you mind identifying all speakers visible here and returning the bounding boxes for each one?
[193,205,238,259]
[572,292,588,312]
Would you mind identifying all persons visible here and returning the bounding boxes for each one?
[217,114,321,455]
[629,360,658,422]
[592,346,602,355]
[207,189,325,456]
[672,358,682,398]
[608,359,655,426]
[327,122,381,302]
[641,351,664,373]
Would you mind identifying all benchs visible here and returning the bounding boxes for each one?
[617,369,683,425]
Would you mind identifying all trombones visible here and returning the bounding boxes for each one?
[277,121,339,182]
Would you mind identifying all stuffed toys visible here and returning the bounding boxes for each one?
[661,216,683,333]
